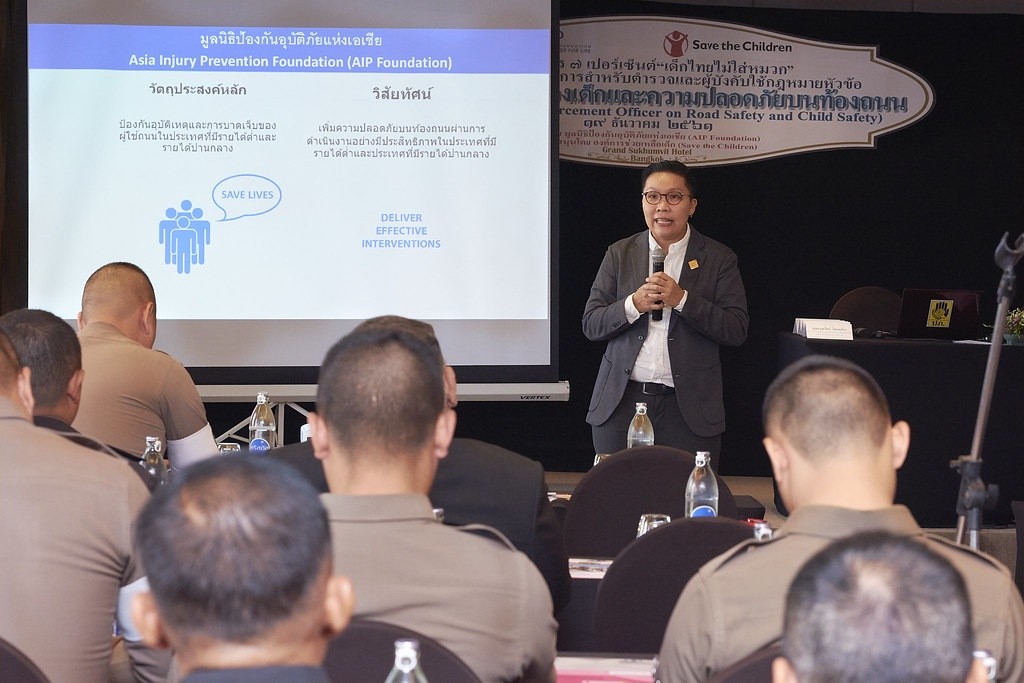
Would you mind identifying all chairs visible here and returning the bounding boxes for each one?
[828,287,905,337]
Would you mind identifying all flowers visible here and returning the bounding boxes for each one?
[1004,308,1024,337]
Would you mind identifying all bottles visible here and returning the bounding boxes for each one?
[384,639,428,683]
[685,450,719,519]
[249,391,275,450]
[140,436,166,486]
[628,403,654,448]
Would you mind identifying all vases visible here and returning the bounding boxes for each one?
[1007,333,1023,347]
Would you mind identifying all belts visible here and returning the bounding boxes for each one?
[625,378,676,396]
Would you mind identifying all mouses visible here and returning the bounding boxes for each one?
[870,330,884,339]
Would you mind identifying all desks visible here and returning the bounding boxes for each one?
[774,329,1022,526]
[542,471,774,510]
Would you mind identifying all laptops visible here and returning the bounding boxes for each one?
[882,288,987,339]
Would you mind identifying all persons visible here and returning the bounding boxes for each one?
[656,358,1024,683]
[771,529,988,683]
[265,314,570,614]
[582,160,750,473]
[132,452,352,683]
[0,330,174,683]
[0,310,171,469]
[71,262,219,470]
[309,329,559,683]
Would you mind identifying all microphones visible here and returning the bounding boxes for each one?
[651,248,668,321]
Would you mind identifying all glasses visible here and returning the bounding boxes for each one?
[640,190,692,204]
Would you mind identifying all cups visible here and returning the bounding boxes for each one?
[217,443,241,455]
[636,514,671,538]
[594,454,612,466]
